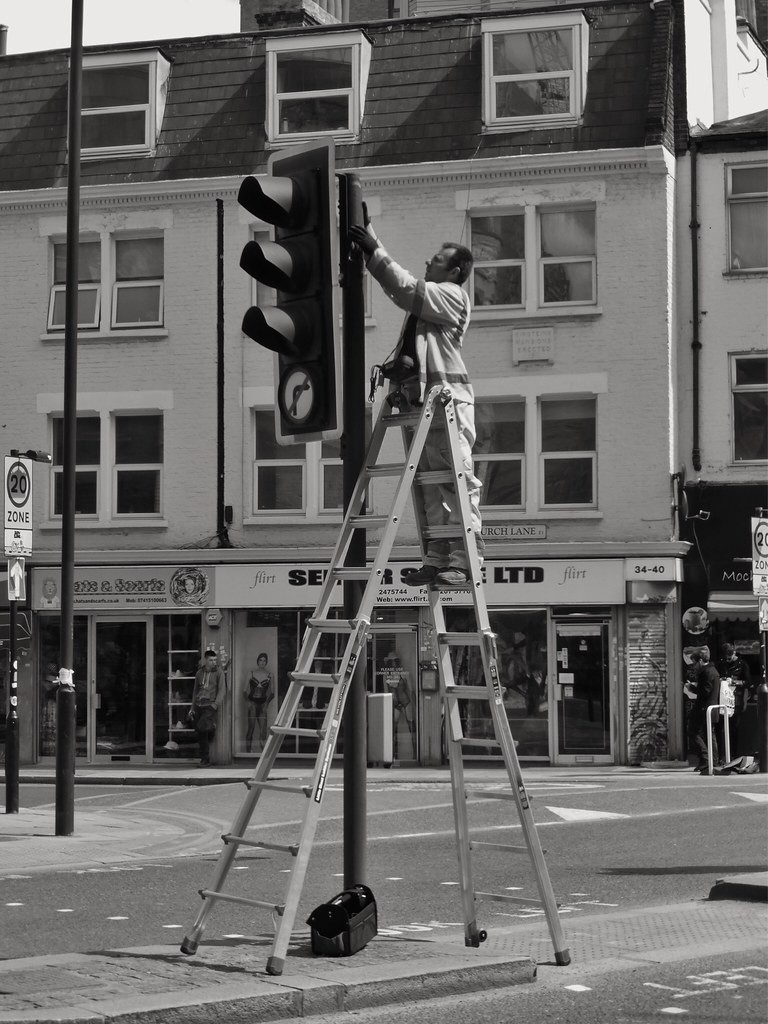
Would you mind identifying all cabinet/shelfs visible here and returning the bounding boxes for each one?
[168,615,200,741]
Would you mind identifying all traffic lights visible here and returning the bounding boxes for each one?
[233,134,348,441]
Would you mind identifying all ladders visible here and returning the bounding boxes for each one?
[183,388,576,975]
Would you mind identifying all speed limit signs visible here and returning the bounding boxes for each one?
[754,521,768,557]
[7,461,30,507]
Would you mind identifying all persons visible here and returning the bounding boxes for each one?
[242,654,274,752]
[184,650,224,768]
[681,643,758,775]
[348,201,486,585]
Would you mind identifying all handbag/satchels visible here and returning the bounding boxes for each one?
[719,678,736,717]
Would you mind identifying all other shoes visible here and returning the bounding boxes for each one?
[694,764,707,772]
[199,760,210,767]
[209,723,216,740]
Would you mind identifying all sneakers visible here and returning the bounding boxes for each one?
[435,566,470,585]
[404,565,441,586]
